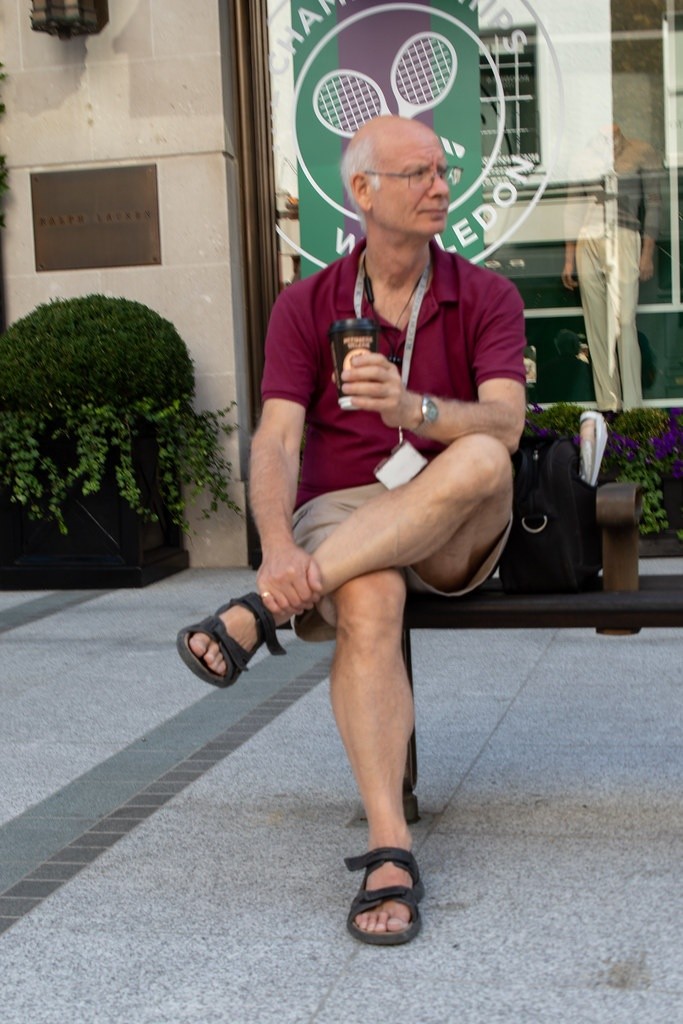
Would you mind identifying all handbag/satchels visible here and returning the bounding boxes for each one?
[500,439,604,592]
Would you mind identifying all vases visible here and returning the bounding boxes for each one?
[662,475,683,529]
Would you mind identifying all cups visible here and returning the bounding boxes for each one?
[326,318,383,412]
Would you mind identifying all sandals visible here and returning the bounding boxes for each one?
[343,847,426,944]
[176,591,287,688]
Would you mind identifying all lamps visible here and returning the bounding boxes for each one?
[29,0,109,41]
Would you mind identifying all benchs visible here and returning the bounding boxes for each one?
[291,479,683,822]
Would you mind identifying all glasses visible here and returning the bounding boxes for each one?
[365,166,464,191]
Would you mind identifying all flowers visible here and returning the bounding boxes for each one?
[522,403,683,541]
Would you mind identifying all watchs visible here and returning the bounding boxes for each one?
[412,392,439,434]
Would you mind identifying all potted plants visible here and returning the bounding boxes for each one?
[0,294,242,590]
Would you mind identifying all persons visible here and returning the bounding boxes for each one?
[175,117,529,945]
[562,96,660,414]
